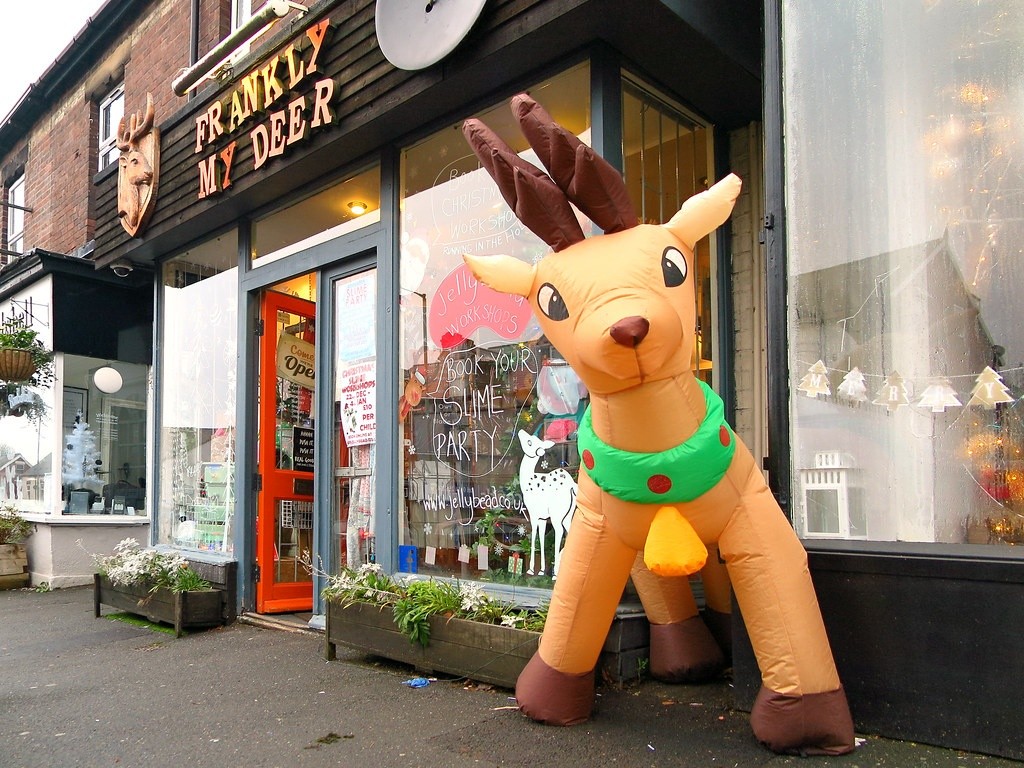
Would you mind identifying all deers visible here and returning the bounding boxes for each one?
[115,92,156,227]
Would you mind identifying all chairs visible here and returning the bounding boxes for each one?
[103,484,138,510]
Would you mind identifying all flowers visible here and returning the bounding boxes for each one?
[75,537,211,594]
[296,547,550,645]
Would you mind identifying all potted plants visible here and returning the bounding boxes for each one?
[0,316,59,389]
[0,500,34,576]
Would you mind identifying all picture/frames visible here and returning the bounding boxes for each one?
[63,385,90,428]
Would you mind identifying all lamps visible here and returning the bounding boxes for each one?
[170,0,310,98]
[93,367,123,394]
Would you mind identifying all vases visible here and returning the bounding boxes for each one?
[90,572,222,638]
[321,593,543,688]
